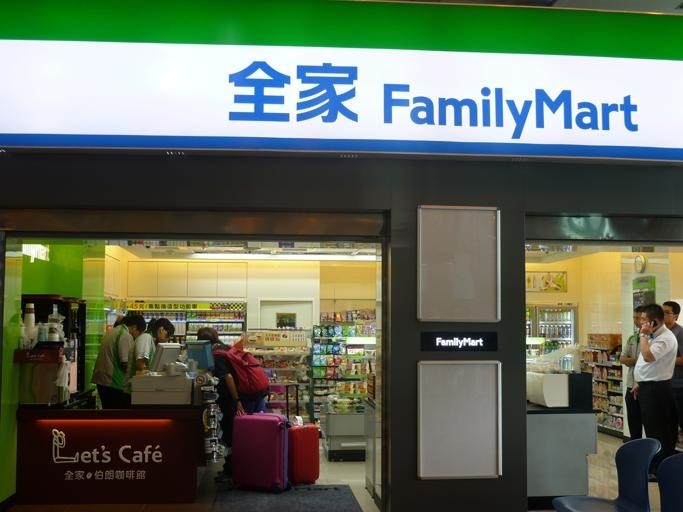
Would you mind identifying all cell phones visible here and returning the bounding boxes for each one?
[650,320,657,327]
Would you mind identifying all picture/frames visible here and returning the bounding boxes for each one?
[634,255,645,273]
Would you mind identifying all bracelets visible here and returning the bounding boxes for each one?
[638,331,649,339]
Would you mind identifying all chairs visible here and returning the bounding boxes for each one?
[660,451,681,512]
[550,436,659,512]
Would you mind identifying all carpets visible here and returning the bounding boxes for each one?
[211,482,361,512]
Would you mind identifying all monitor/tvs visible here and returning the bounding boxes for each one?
[149,342,181,371]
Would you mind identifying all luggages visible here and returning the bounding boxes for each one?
[232,413,293,496]
[286,422,321,484]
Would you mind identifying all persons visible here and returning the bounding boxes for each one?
[91,313,268,481]
[661,301,683,433]
[619,306,653,441]
[627,304,677,482]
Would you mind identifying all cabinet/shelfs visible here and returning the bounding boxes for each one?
[524,303,623,437]
[244,327,312,426]
[128,301,189,347]
[313,312,372,462]
[127,258,246,301]
[187,301,248,348]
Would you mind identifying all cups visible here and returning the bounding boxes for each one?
[37,323,48,342]
[23,303,35,341]
[47,323,59,342]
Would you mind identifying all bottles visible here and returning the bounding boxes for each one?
[525,307,529,358]
[65,303,82,353]
[538,308,570,372]
[18,323,25,351]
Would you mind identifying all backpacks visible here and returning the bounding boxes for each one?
[217,348,271,402]
